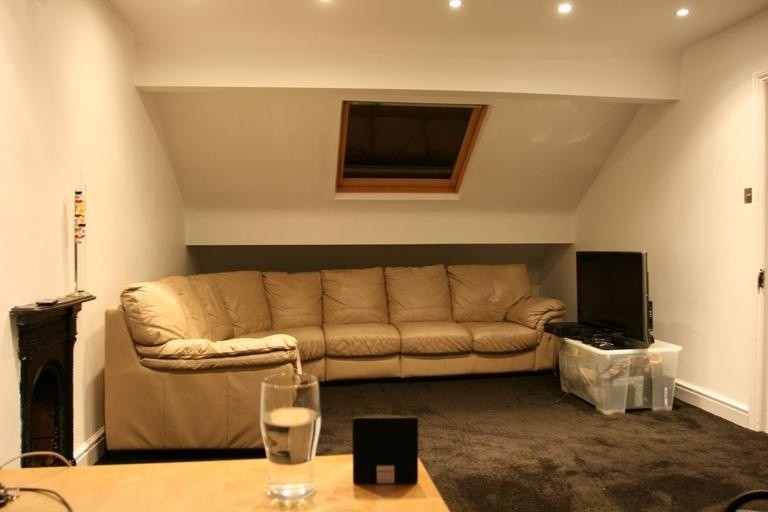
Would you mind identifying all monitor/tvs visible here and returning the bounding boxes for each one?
[576,249,654,350]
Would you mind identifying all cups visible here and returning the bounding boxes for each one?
[259,371,322,505]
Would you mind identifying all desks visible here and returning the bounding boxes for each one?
[0,450,456,512]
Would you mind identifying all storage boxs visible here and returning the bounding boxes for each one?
[554,333,685,418]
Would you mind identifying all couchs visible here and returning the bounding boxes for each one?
[103,261,569,459]
